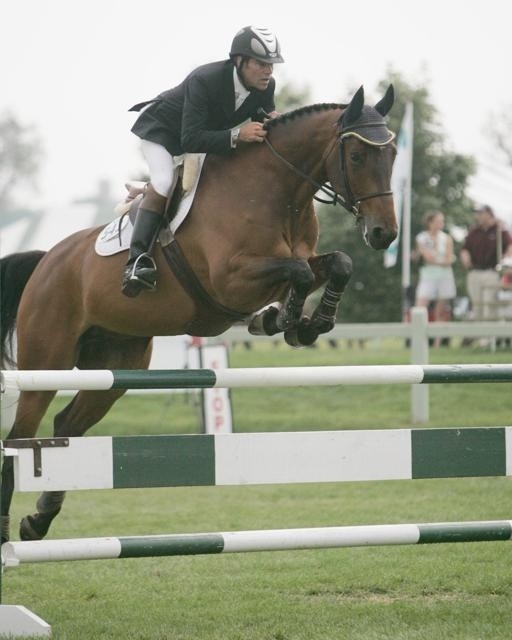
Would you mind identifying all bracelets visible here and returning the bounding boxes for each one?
[233,127,238,145]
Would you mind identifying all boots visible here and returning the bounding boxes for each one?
[121,207,162,298]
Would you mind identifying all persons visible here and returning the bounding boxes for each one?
[121,26,285,298]
[416,205,512,348]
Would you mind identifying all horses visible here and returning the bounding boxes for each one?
[0,82,400,543]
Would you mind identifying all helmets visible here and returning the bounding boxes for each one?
[229,23,284,63]
